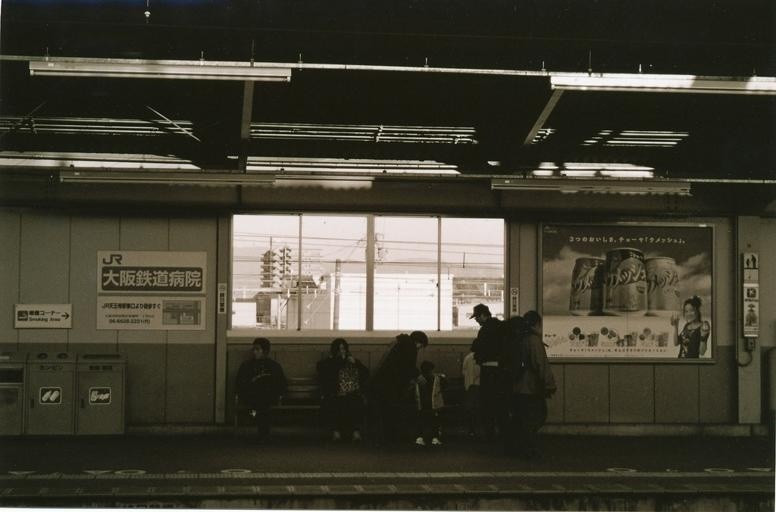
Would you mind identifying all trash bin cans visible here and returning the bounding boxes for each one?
[0,350,129,438]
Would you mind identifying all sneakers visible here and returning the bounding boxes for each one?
[331,429,445,451]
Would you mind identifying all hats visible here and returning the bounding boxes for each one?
[468,303,492,321]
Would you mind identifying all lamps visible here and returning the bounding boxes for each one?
[548,71,776,95]
[25,58,294,83]
[55,167,375,186]
[488,177,694,195]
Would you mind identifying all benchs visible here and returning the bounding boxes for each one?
[232,372,367,439]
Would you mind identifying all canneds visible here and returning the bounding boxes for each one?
[645,256,683,312]
[568,257,606,317]
[604,247,648,313]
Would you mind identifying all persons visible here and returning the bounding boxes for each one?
[409,362,450,448]
[468,304,557,458]
[375,331,429,446]
[236,337,286,444]
[319,337,369,450]
[672,296,710,358]
